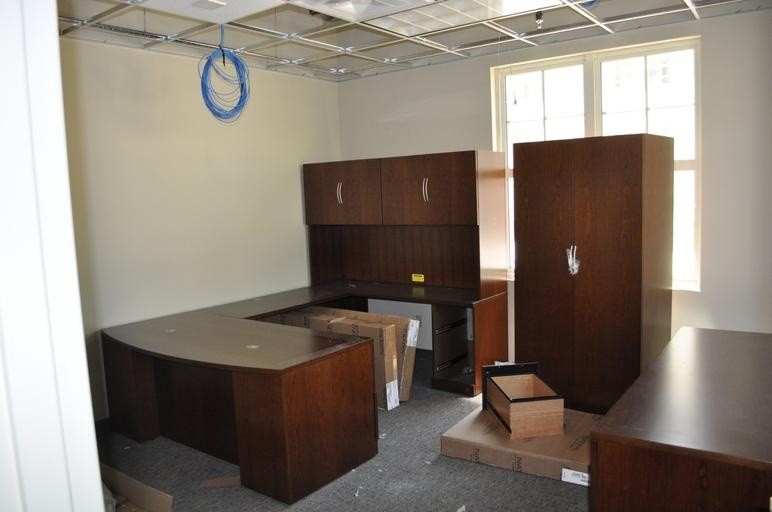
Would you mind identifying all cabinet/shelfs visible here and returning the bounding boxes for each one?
[381,150,506,226]
[513,133,674,415]
[589,326,772,512]
[302,158,383,226]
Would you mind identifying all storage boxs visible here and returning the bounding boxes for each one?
[485,373,565,440]
[441,407,604,486]
[101,462,173,512]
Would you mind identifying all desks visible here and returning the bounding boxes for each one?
[102,281,507,507]
